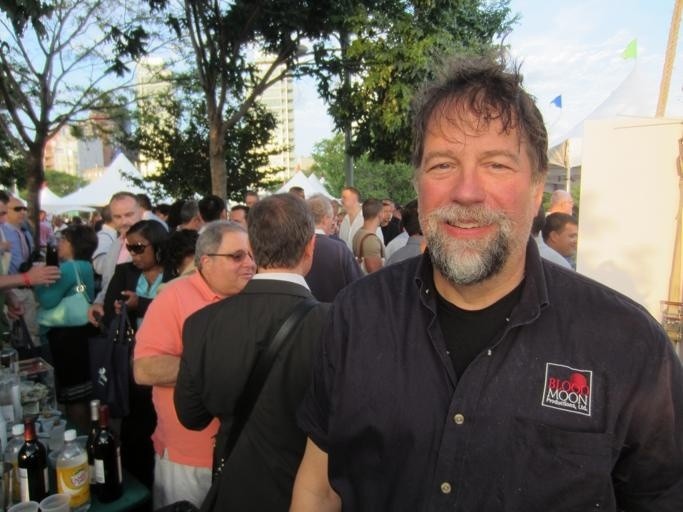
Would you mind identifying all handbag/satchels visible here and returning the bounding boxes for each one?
[90,302,136,417]
[35,286,92,329]
[354,255,387,275]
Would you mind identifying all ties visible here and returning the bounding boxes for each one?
[17,229,29,265]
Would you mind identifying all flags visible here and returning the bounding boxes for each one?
[621,40,637,58]
[551,94,561,108]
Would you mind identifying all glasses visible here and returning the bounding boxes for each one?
[203,251,253,262]
[125,242,151,255]
[9,207,28,212]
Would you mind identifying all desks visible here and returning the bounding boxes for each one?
[91,469,152,512]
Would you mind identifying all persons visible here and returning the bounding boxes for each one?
[1,61,683,512]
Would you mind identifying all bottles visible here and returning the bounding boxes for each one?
[0,306,124,512]
[46,231,62,267]
